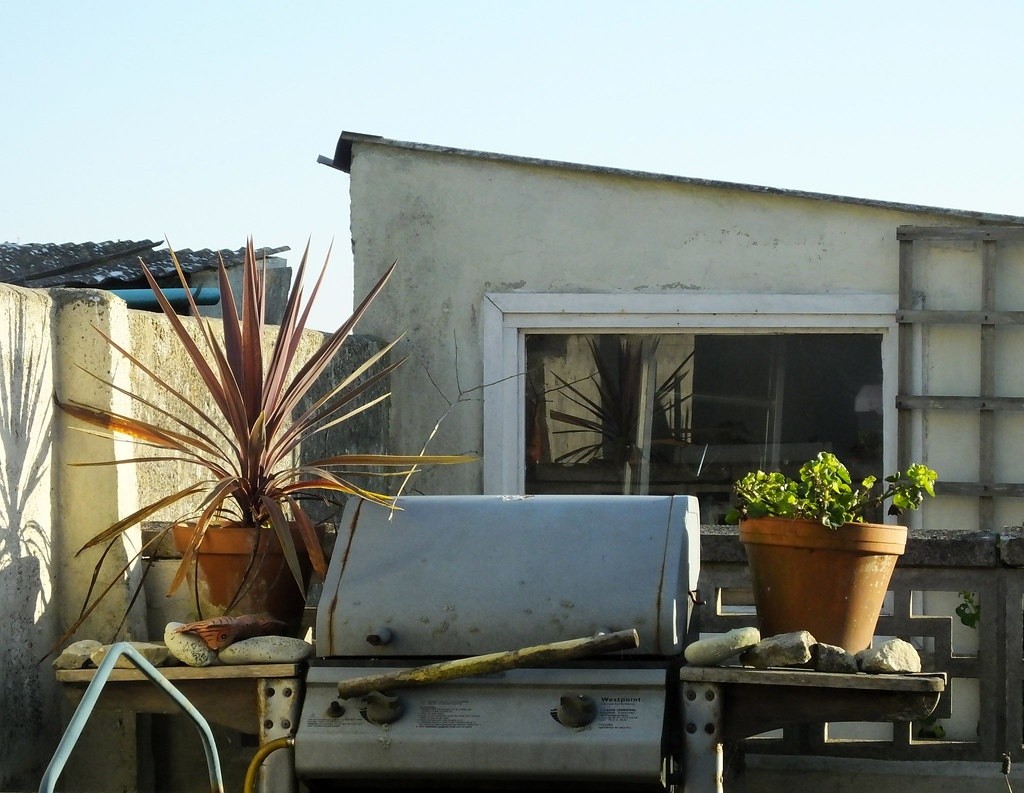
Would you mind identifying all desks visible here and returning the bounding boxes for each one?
[676,664,943,793]
[53,664,305,793]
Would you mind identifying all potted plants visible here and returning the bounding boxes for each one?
[52,231,482,637]
[724,450,937,655]
